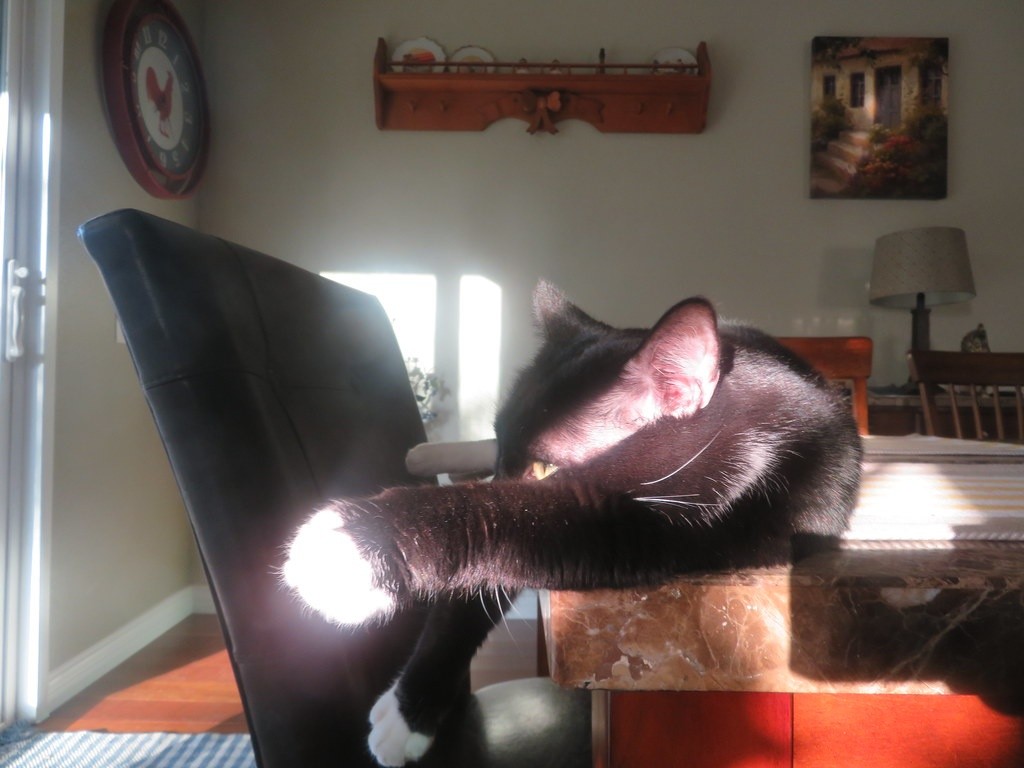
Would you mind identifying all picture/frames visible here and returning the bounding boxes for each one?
[807,33,950,202]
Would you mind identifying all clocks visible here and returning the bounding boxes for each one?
[102,0,212,201]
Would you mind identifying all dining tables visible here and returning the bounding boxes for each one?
[526,431,1024,768]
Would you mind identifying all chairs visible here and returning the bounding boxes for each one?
[76,203,594,768]
[907,348,1023,440]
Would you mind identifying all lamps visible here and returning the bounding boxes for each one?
[866,227,977,401]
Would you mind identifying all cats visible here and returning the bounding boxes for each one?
[274,278,864,768]
